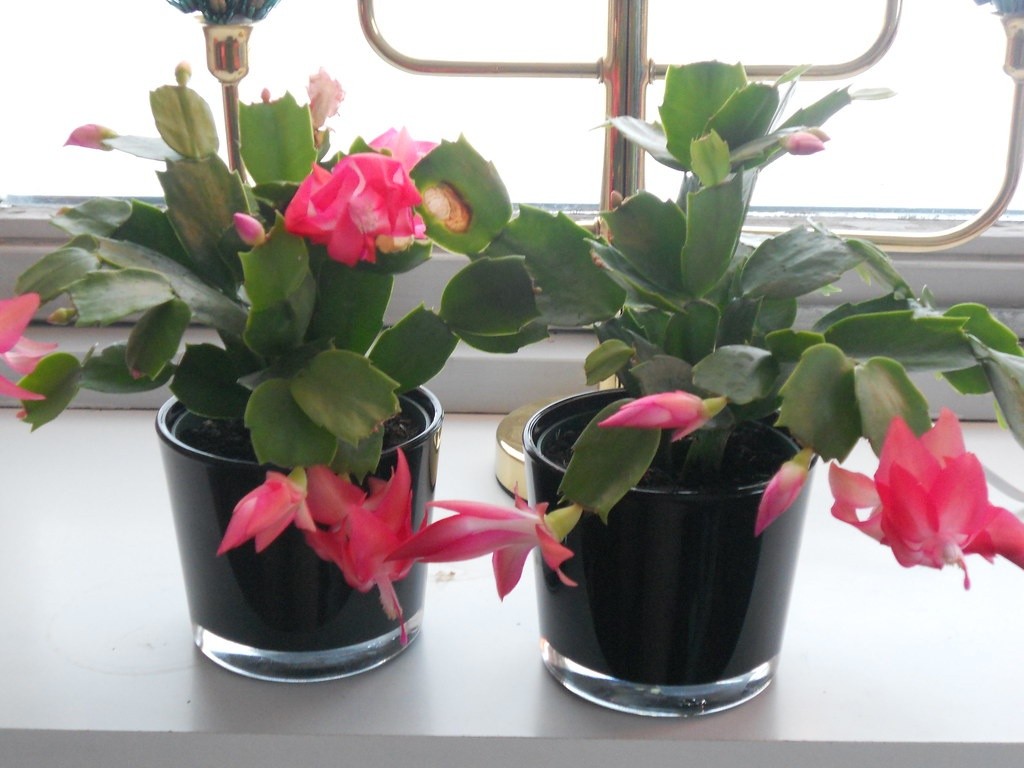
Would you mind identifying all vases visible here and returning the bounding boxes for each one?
[517,384,817,722]
[143,357,441,689]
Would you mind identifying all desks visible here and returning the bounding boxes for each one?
[0,415,1024,750]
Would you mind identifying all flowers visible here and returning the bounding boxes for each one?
[385,60,1020,609]
[0,73,453,615]
